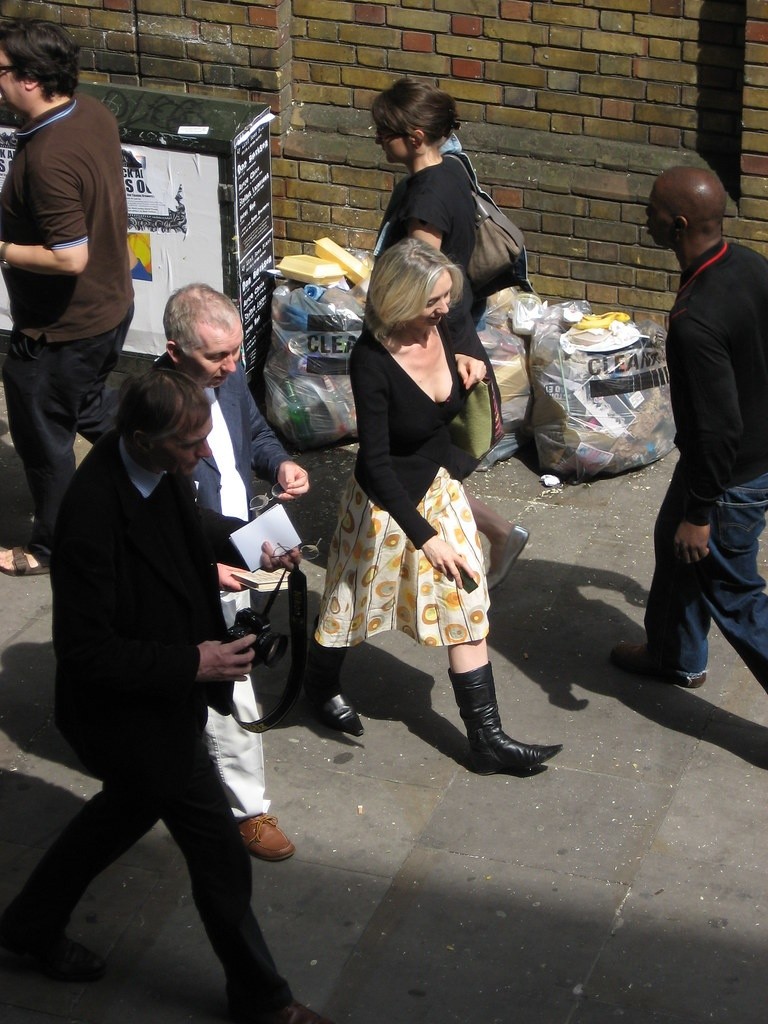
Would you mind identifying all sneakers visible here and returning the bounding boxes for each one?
[610,642,706,689]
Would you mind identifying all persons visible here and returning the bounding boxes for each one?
[0,368,331,1024]
[152,283,310,863]
[611,164,767,695]
[0,18,135,577]
[303,237,563,776]
[369,78,530,589]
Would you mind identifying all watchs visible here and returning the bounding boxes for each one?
[0,242,13,269]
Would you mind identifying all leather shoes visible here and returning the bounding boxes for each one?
[231,996,335,1024]
[0,919,114,981]
[486,523,530,590]
[238,813,297,863]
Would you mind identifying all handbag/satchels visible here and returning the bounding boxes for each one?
[452,381,493,459]
[445,151,525,290]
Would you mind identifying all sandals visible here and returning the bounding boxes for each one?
[0,546,49,577]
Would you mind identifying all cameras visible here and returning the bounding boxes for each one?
[223,608,289,668]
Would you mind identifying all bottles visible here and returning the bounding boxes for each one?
[322,375,356,433]
[283,379,316,444]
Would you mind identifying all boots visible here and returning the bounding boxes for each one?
[448,662,562,776]
[303,626,366,737]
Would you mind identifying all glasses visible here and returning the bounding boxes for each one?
[249,482,287,512]
[270,537,324,560]
